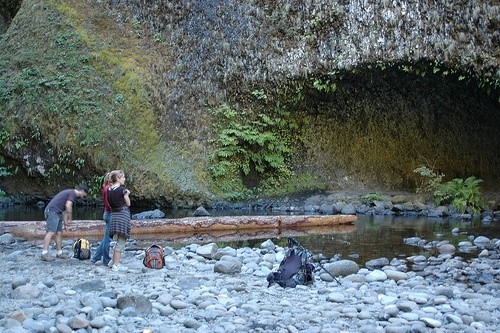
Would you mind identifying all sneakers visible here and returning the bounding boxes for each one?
[41,252,55,261]
[108,259,128,271]
[56,252,68,258]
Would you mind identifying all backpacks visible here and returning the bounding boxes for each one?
[267,248,315,290]
[73,238,91,260]
[143,244,164,268]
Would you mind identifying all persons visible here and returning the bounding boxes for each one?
[89,172,113,266]
[41,184,88,261]
[107,170,130,271]
